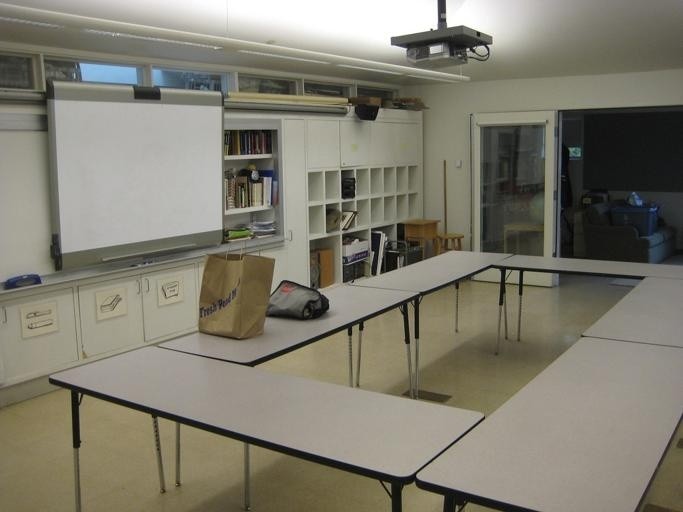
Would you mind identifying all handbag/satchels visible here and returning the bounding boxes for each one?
[199,237,329,340]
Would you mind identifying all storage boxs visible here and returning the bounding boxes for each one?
[611,205,658,236]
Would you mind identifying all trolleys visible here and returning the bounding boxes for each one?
[382,236,426,272]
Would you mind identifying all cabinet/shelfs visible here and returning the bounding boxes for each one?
[0,89,425,409]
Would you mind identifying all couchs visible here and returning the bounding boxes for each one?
[583,200,676,264]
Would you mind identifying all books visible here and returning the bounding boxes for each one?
[342,178,355,198]
[341,211,356,231]
[221,128,279,244]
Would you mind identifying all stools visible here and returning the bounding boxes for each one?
[437,233,464,255]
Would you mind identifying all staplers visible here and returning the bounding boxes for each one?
[5,274,42,288]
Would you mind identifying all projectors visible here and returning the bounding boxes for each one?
[406,42,468,70]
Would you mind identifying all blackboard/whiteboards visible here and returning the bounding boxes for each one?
[47,81,224,271]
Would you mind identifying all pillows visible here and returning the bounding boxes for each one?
[586,202,611,226]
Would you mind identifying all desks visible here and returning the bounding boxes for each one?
[406,219,441,261]
[48,250,683,511]
[502,222,544,253]
